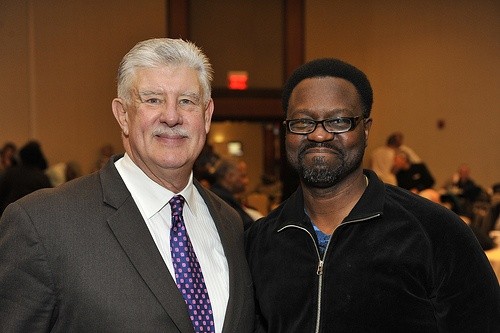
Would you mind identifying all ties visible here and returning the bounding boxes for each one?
[168,194,215,333]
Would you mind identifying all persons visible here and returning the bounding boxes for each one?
[245,57,500,333]
[0,130,499,251]
[0,38,257,333]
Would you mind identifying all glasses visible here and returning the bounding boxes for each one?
[286,117,356,135]
